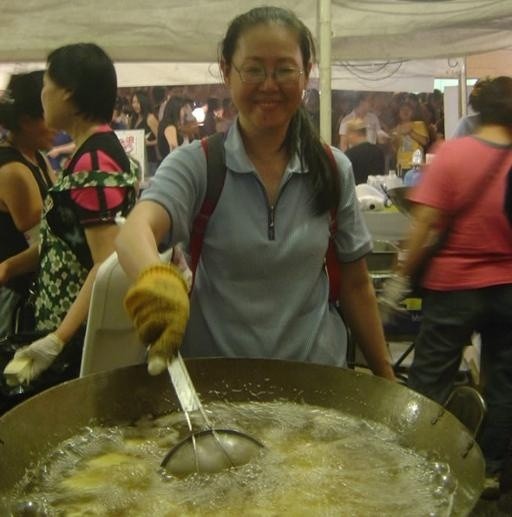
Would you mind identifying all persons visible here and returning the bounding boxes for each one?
[0,42,143,407]
[47,131,76,173]
[375,75,512,501]
[303,85,485,186]
[110,2,396,388]
[114,90,237,168]
[1,68,62,358]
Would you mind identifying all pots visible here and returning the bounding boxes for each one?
[1,356,490,517]
[380,184,422,224]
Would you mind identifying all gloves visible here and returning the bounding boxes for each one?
[382,271,412,300]
[126,267,189,376]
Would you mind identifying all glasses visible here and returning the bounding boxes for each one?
[231,64,304,85]
[7,333,63,388]
